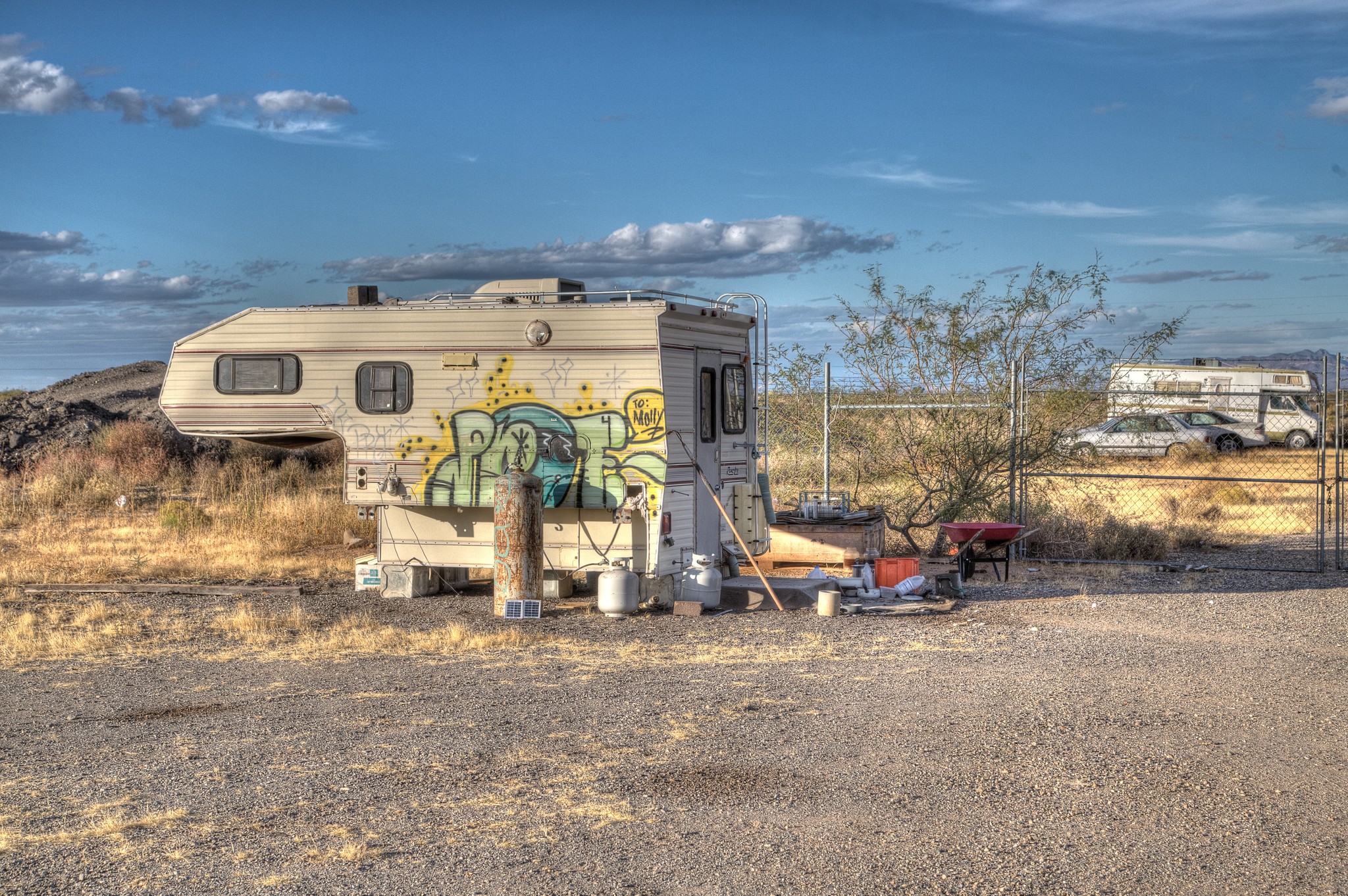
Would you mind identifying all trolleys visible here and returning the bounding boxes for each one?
[938,522,1041,583]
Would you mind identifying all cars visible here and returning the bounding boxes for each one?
[1049,412,1219,463]
[1162,409,1270,454]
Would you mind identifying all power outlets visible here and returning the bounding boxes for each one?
[387,463,396,473]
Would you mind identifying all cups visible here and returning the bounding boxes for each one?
[818,591,841,616]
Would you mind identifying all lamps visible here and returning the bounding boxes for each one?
[388,477,397,496]
[377,476,388,494]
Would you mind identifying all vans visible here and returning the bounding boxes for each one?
[1106,357,1325,451]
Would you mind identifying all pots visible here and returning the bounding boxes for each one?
[857,585,880,598]
[840,603,862,613]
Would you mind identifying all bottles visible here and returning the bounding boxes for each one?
[893,576,926,598]
[807,566,827,578]
[863,548,880,564]
[648,595,659,605]
[843,547,859,570]
[802,501,846,517]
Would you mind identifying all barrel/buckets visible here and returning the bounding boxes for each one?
[851,564,875,578]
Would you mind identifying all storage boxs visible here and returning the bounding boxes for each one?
[875,557,921,594]
[354,564,381,592]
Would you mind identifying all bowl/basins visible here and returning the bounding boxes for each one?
[842,589,857,597]
[841,510,869,519]
